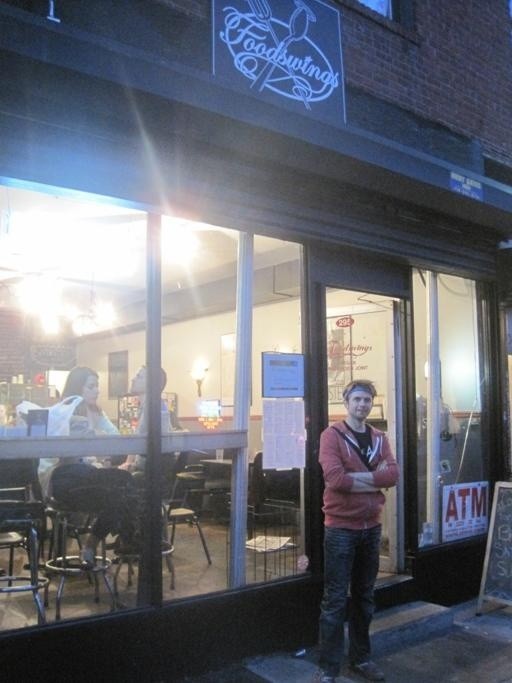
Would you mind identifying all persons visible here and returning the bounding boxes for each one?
[124,362,180,480]
[317,378,401,682]
[42,364,177,572]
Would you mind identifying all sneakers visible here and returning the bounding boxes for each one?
[350,657,385,681]
[80,545,96,570]
[312,660,340,683]
[121,540,174,558]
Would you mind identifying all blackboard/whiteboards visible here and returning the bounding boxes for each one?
[479,481,512,609]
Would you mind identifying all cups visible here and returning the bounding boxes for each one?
[119,417,131,435]
